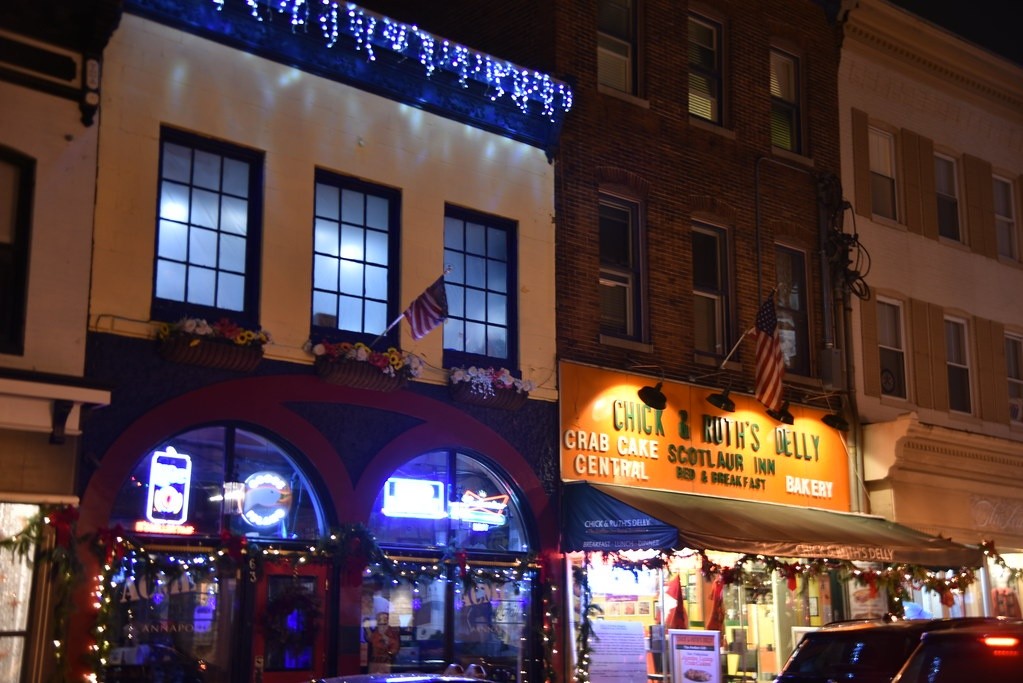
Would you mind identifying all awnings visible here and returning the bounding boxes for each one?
[559,478,985,571]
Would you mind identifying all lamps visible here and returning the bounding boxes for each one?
[624,361,667,410]
[688,372,735,412]
[746,383,794,425]
[801,394,849,432]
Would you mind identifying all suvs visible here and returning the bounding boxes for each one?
[773,617,1022,682]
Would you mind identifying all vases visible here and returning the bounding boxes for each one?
[313,358,405,391]
[159,331,263,373]
[450,381,527,411]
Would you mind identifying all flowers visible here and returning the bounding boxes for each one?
[157,314,272,348]
[304,338,424,377]
[449,366,540,400]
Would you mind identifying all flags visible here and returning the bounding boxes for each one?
[752,288,787,414]
[403,275,449,341]
